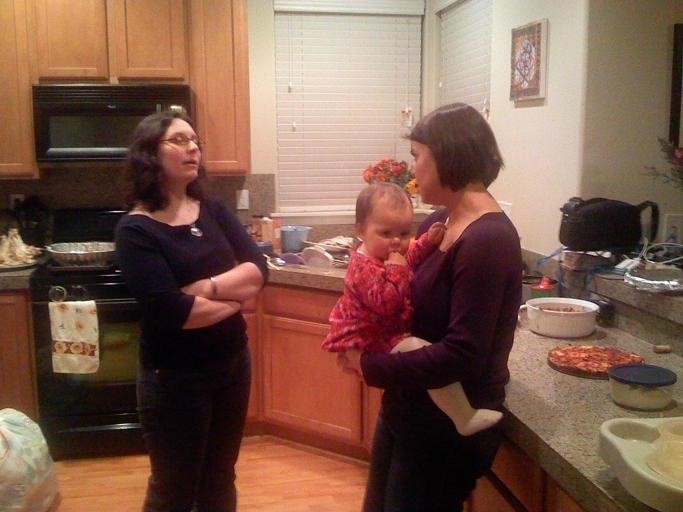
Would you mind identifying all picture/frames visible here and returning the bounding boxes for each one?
[506,18,547,101]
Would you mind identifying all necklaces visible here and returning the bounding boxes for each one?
[166,195,203,239]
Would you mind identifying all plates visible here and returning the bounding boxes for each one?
[43,260,115,272]
[279,239,351,269]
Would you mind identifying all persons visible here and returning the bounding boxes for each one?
[317,179,504,439]
[110,107,269,511]
[334,102,524,512]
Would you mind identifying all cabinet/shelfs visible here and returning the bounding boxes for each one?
[361,376,382,440]
[538,466,585,511]
[261,283,362,437]
[0,293,39,422]
[188,0,250,181]
[465,437,538,511]
[0,1,33,182]
[236,283,261,421]
[33,1,188,84]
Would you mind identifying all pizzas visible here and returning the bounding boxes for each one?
[547,345,645,376]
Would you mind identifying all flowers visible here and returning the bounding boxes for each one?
[361,157,419,195]
[637,135,682,195]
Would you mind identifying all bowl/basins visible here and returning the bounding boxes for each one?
[40,241,124,265]
[279,224,311,252]
[525,295,601,338]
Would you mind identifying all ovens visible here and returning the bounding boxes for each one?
[32,299,148,460]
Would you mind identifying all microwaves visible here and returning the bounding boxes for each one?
[30,83,195,164]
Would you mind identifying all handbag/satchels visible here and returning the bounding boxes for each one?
[559,195,660,250]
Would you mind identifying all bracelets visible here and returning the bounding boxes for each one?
[208,273,219,300]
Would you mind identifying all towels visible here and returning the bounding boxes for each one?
[47,300,102,375]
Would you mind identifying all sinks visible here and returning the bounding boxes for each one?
[274,242,359,271]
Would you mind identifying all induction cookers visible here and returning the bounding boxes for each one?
[29,257,137,306]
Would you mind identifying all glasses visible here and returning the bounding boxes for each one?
[155,136,206,146]
